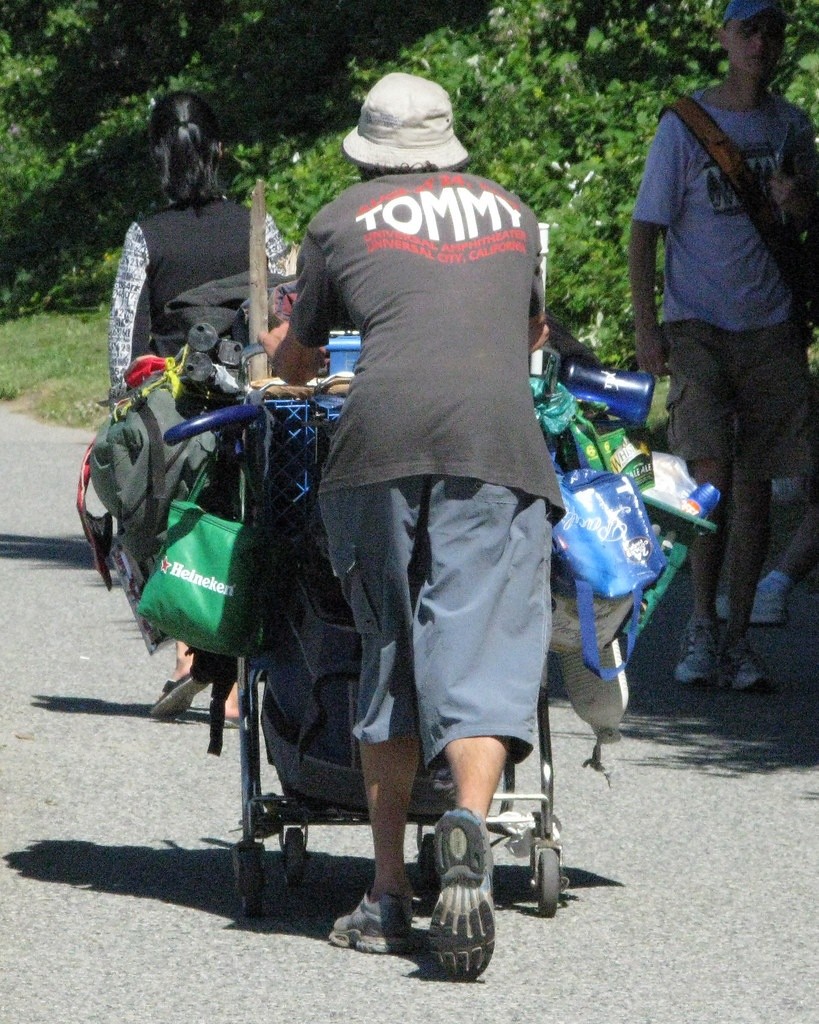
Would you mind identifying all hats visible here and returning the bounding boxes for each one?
[342,72,471,171]
[723,0,792,28]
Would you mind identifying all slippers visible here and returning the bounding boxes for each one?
[224,718,240,729]
[150,673,210,717]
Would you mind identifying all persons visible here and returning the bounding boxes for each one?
[716,502,819,624]
[628,1,819,691]
[258,70,550,983]
[106,93,290,725]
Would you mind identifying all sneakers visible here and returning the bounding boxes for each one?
[329,885,423,953]
[673,620,721,685]
[714,592,790,625]
[717,639,774,690]
[428,808,495,981]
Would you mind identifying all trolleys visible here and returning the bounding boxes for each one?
[161,327,571,918]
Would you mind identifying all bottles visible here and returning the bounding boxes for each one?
[558,359,655,426]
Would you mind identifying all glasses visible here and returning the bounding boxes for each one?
[725,21,785,42]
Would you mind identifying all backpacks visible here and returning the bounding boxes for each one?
[548,436,668,682]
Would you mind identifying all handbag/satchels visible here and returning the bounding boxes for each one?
[135,457,264,658]
[569,413,655,492]
[90,388,217,563]
[784,222,819,329]
